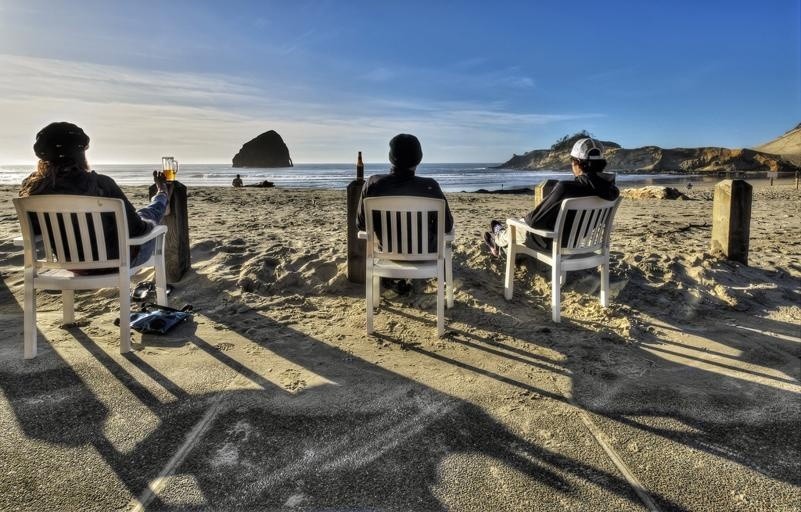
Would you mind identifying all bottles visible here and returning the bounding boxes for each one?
[356,151,364,181]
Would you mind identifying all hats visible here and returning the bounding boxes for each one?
[34,122,90,160]
[571,138,604,159]
[388,134,423,167]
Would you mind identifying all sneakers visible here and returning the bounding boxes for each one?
[483,232,498,256]
[491,220,504,235]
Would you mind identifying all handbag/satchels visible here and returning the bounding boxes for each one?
[128,301,193,336]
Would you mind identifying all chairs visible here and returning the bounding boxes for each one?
[13,194,168,361]
[357,196,456,337]
[504,196,624,324]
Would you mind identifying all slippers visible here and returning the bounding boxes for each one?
[155,283,175,297]
[133,280,153,302]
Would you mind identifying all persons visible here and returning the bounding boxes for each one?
[481,137,618,257]
[20,122,174,273]
[232,174,244,187]
[354,132,454,293]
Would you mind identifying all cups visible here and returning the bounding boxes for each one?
[160,155,179,182]
